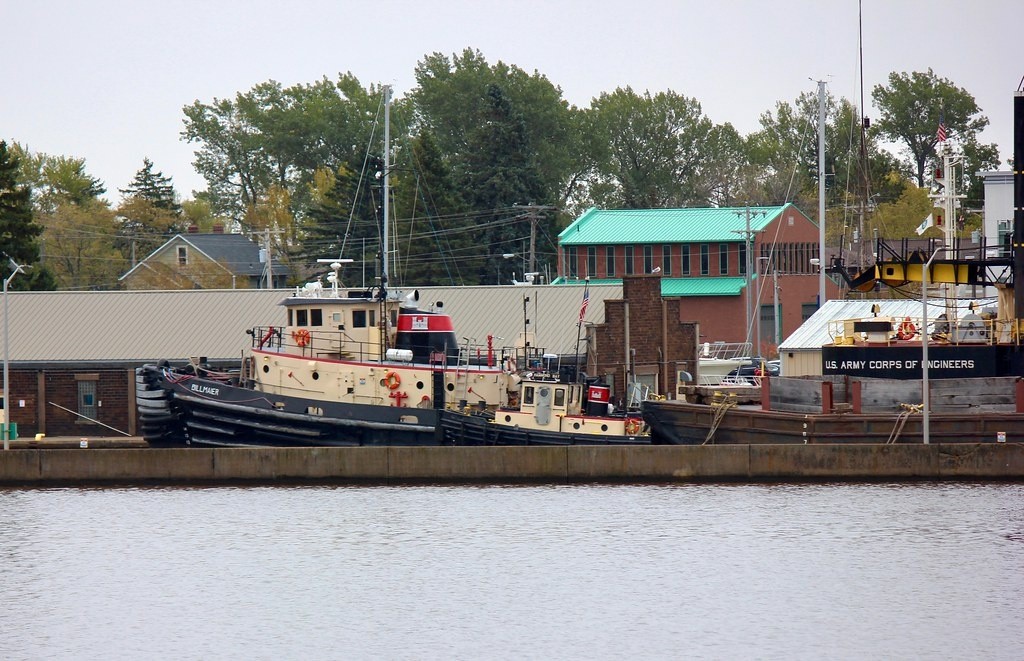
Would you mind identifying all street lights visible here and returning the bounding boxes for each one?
[501,253,536,273]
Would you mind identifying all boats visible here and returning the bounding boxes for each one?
[130,84,654,451]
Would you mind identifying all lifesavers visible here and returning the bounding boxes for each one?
[296,328,310,347]
[623,419,639,434]
[898,321,916,340]
[384,372,401,389]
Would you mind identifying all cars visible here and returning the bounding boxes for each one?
[729,353,781,386]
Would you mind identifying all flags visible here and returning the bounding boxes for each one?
[915,212,933,236]
[579,287,589,322]
[937,109,947,142]
[958,215,966,232]
